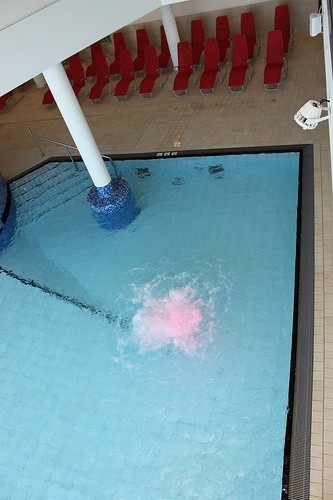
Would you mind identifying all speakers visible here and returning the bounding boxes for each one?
[309,12,322,37]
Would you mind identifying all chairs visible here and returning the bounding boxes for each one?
[67,53,81,79]
[263,30,286,92]
[216,17,231,66]
[110,32,127,75]
[173,41,194,96]
[86,43,103,76]
[114,49,137,102]
[200,40,223,95]
[275,4,294,55]
[73,63,85,96]
[43,91,53,104]
[240,12,260,59]
[190,19,208,67]
[228,35,253,94]
[139,46,162,99]
[89,54,111,103]
[158,25,172,68]
[133,29,150,74]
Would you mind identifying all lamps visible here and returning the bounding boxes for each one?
[294,99,321,130]
[310,12,321,36]
